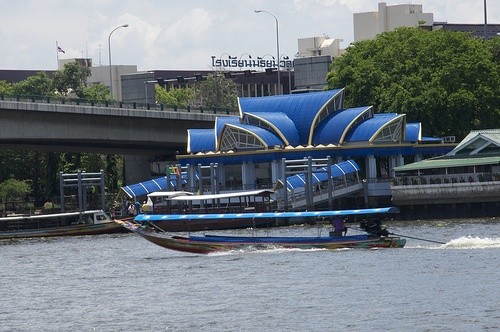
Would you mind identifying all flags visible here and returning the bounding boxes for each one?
[58,46,65,54]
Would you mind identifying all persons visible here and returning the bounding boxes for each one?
[460,176,465,182]
[122,203,128,214]
[331,216,347,236]
[105,201,121,219]
[142,201,150,212]
[128,204,135,216]
[391,178,398,186]
[254,180,259,189]
[400,176,409,185]
[435,175,441,182]
[135,200,141,215]
[316,185,324,191]
[128,201,133,209]
[452,175,458,183]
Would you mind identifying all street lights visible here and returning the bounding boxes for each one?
[108,25,129,108]
[220,53,231,72]
[262,54,275,72]
[239,53,251,71]
[255,10,281,95]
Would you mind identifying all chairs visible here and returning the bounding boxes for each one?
[327,227,342,237]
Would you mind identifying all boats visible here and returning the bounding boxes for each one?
[113,207,407,255]
[0,210,125,239]
[165,189,279,215]
